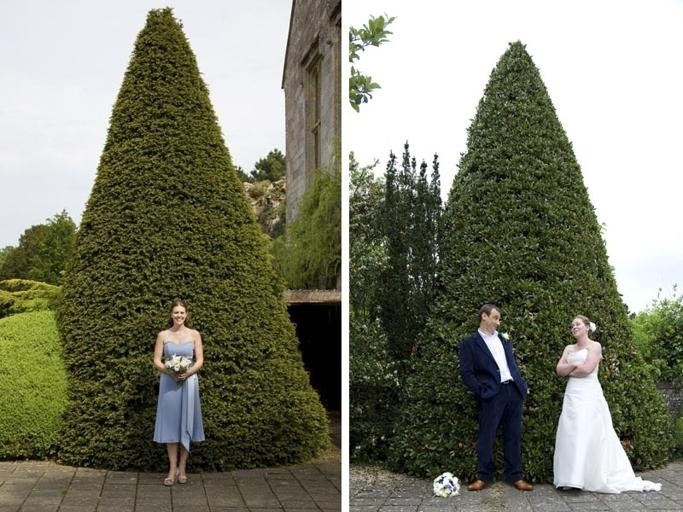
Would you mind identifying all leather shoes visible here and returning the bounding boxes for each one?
[468,480,485,490]
[514,480,533,490]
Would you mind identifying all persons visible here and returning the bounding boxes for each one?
[457,301,535,492]
[151,298,207,486]
[550,312,663,495]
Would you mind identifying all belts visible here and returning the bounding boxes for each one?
[504,380,511,384]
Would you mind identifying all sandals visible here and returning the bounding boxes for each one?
[164,469,187,486]
